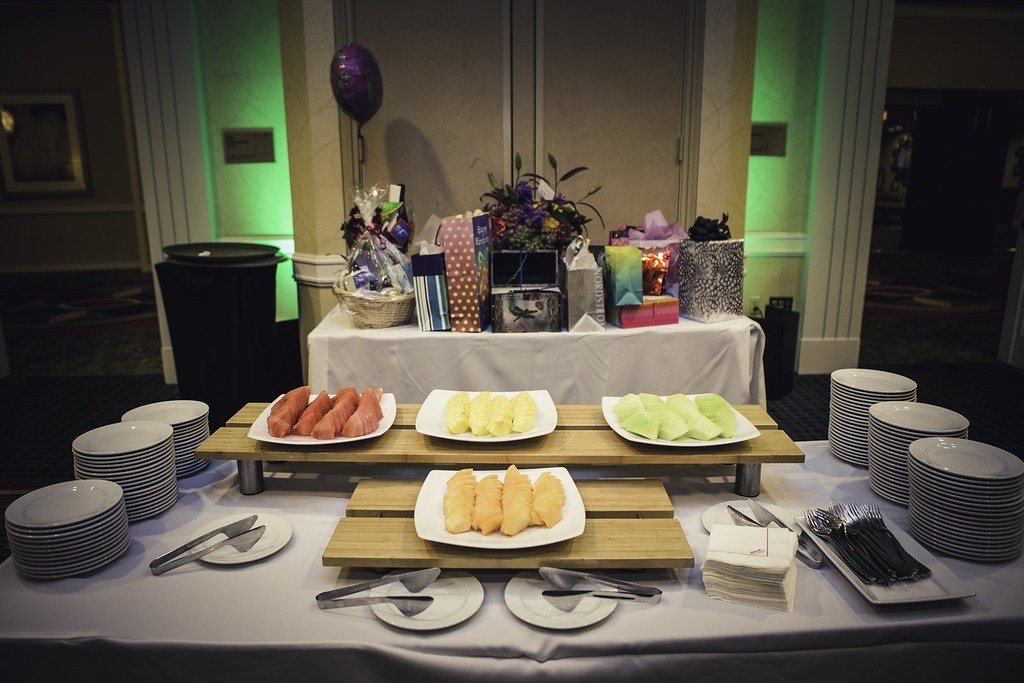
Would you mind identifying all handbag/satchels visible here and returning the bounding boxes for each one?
[411,252,451,332]
[435,209,492,332]
[606,245,644,305]
[564,234,606,332]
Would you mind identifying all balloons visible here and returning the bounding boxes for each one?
[329,41,384,135]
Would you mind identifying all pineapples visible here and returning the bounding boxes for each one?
[444,391,538,437]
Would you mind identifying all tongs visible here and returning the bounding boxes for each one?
[316,568,442,619]
[539,566,662,612]
[148,514,266,577]
[727,498,824,569]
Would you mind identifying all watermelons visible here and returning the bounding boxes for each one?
[266,386,384,440]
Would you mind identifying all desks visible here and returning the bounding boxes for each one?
[0,440,1024,683]
[306,297,767,412]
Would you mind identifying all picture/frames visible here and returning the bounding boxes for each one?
[0,89,95,201]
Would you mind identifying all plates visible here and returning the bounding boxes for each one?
[503,570,618,629]
[5,397,209,581]
[827,367,1024,562]
[188,513,292,565]
[602,392,762,447]
[700,500,803,539]
[367,568,484,630]
[414,467,586,549]
[793,511,977,606]
[414,388,558,443]
[247,393,396,446]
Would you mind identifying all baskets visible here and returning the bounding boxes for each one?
[332,234,415,328]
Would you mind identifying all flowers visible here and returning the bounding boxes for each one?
[479,152,606,282]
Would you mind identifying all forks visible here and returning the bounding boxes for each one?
[803,497,933,586]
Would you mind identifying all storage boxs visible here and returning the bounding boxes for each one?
[490,248,563,333]
[680,238,744,320]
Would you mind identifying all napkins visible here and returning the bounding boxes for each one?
[700,524,799,614]
